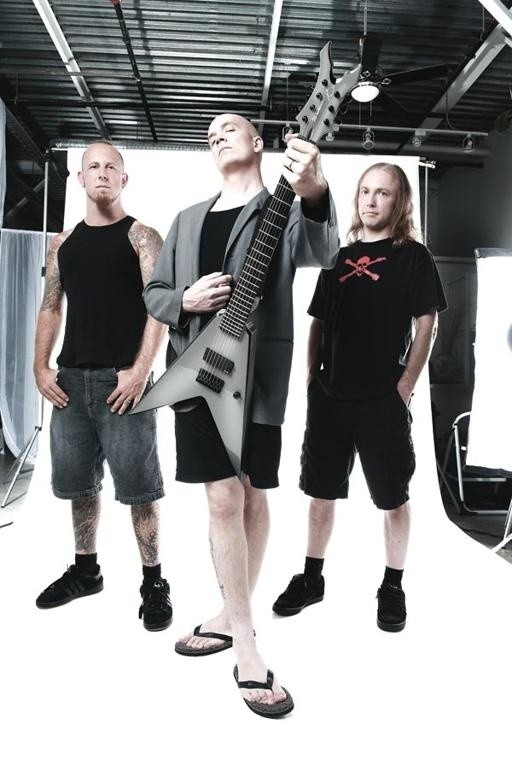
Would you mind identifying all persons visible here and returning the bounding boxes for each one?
[139,110,345,718]
[267,159,449,635]
[25,137,175,636]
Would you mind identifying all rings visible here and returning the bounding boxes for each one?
[285,158,296,172]
[125,398,132,403]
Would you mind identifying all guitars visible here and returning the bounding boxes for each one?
[130,39,364,482]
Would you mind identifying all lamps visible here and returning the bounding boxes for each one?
[348,75,383,104]
[281,120,478,155]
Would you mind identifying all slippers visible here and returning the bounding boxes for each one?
[175,624,256,656]
[233,664,294,718]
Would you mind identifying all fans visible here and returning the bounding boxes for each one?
[289,1,458,122]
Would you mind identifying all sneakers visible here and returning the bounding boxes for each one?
[139,579,172,632]
[376,584,406,632]
[273,574,324,616]
[36,564,103,608]
